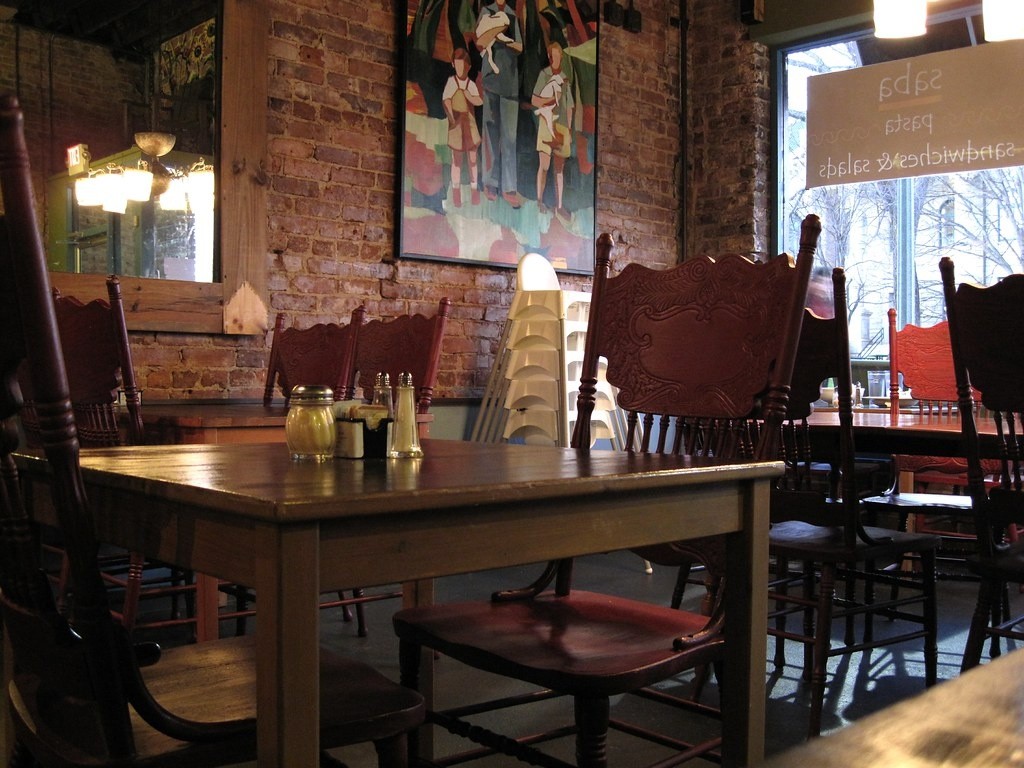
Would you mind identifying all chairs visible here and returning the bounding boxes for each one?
[682,267,936,743]
[0,91,425,768]
[471,252,643,452]
[887,307,1024,580]
[17,274,148,449]
[263,297,451,639]
[939,257,1024,673]
[391,215,817,768]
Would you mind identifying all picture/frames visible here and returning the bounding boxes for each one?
[389,0,601,275]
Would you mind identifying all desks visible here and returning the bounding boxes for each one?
[110,396,434,642]
[16,439,784,768]
[687,410,1024,459]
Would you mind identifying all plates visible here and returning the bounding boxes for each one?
[862,397,919,408]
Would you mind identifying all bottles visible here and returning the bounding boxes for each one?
[832,385,854,408]
[853,381,864,409]
[373,371,394,419]
[286,383,337,461]
[388,371,424,459]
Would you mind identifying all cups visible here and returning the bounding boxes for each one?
[868,370,884,408]
[884,369,904,397]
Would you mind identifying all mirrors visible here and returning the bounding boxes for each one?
[0,0,268,336]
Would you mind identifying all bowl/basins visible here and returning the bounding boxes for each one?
[819,386,865,407]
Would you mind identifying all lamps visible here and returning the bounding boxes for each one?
[75,132,215,215]
[873,0,928,39]
